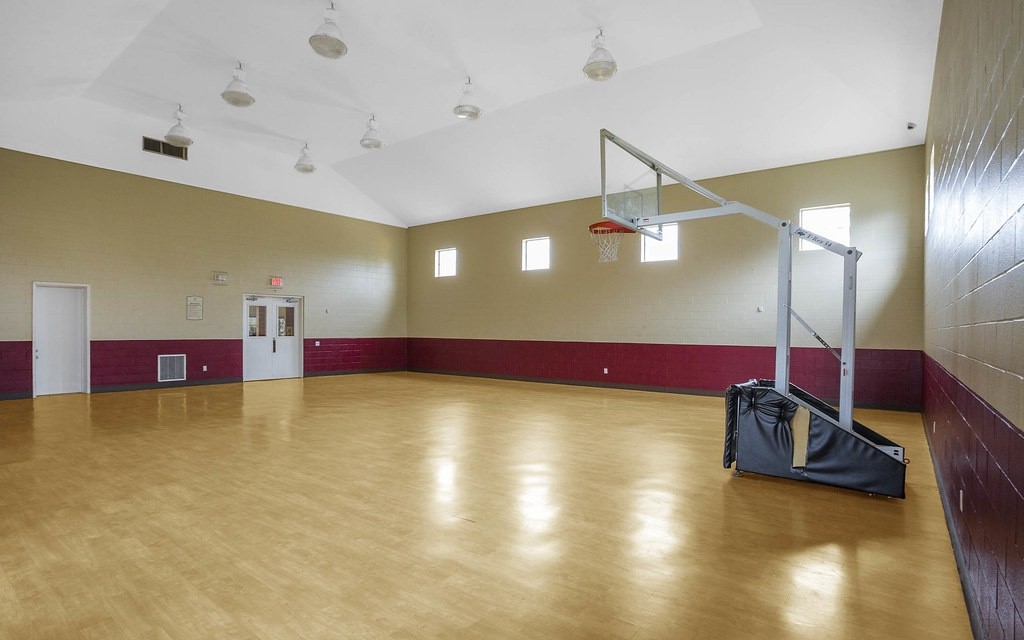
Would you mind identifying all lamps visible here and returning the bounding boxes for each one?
[164,104,194,147]
[452,76,484,120]
[582,29,618,81]
[294,144,317,173]
[359,114,387,149]
[309,0,349,59]
[218,62,256,107]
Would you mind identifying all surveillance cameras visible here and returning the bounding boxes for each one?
[907,122,917,130]
